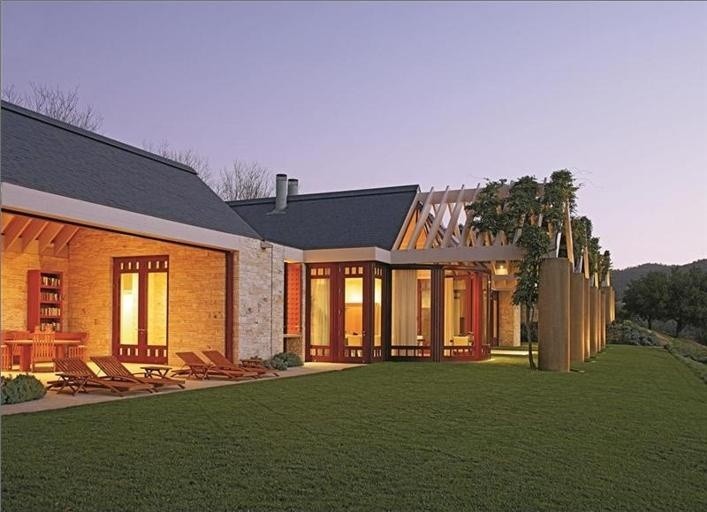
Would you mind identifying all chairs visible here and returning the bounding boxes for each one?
[417,338,426,347]
[0,330,89,372]
[39,355,158,398]
[451,334,470,352]
[85,352,187,394]
[168,348,261,383]
[201,346,281,379]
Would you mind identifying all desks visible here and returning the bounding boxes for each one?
[236,356,259,365]
[136,364,173,379]
[417,336,423,346]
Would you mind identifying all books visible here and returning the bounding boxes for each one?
[40,276,61,331]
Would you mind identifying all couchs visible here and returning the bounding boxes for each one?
[347,333,380,347]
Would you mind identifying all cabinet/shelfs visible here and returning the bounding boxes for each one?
[27,269,64,332]
